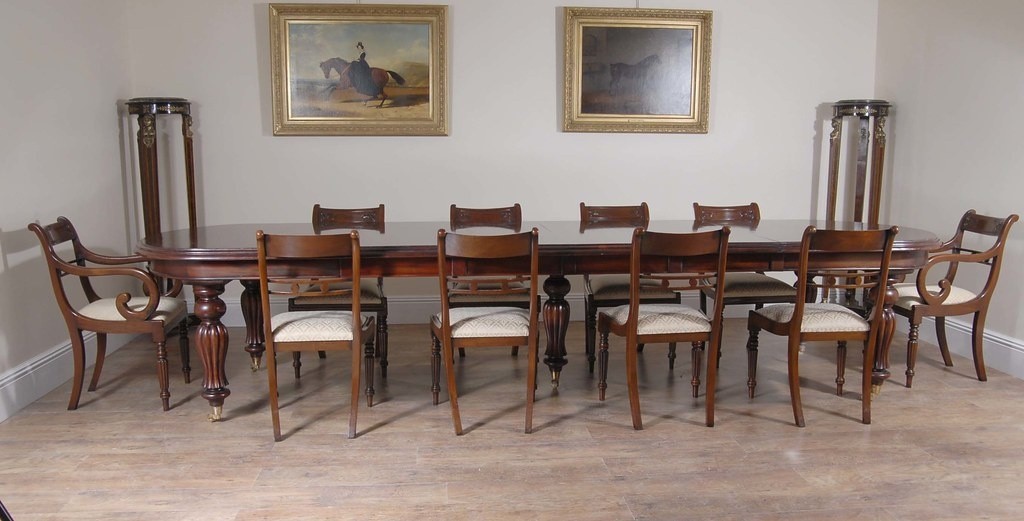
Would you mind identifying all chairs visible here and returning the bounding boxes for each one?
[446,202,541,358]
[27,215,190,415]
[254,228,375,444]
[596,224,731,431]
[287,203,391,380]
[745,222,898,426]
[428,226,542,435]
[866,209,1020,384]
[579,202,682,374]
[691,202,800,372]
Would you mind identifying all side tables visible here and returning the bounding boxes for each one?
[125,95,206,331]
[821,97,892,317]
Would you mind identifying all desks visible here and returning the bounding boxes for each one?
[134,217,947,425]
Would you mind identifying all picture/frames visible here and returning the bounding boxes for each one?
[562,5,714,136]
[268,3,450,137]
